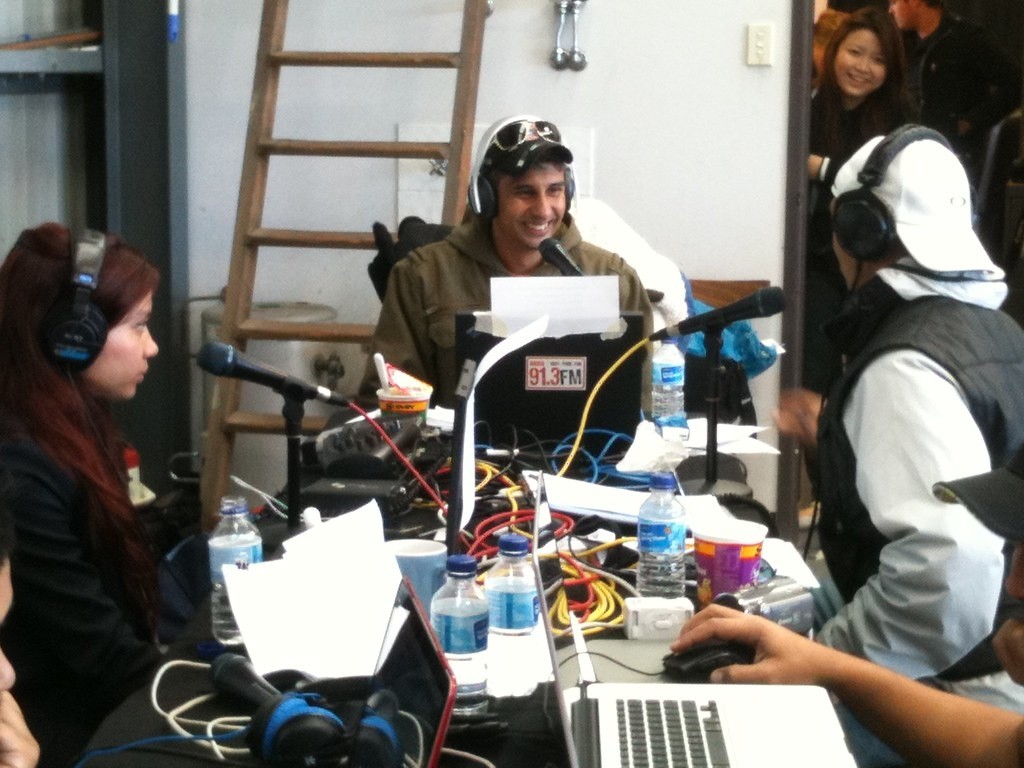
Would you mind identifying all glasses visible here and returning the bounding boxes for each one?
[494,121,562,150]
[752,557,777,588]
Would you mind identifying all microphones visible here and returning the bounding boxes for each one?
[212,653,348,768]
[538,237,586,278]
[350,704,404,768]
[649,286,790,341]
[196,341,353,408]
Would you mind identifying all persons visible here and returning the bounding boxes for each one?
[0,222,160,768]
[671,440,1024,768]
[0,508,41,768]
[803,1,1023,394]
[358,114,653,421]
[816,126,1024,768]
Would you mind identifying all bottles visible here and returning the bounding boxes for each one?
[431,556,488,716]
[637,474,686,598]
[209,494,262,645]
[652,337,690,444]
[485,534,539,699]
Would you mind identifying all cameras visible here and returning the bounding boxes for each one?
[712,578,815,636]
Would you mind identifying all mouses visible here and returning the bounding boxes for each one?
[661,642,753,683]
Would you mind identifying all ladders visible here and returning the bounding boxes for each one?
[200,0,494,533]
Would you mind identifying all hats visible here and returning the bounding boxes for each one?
[832,133,997,270]
[933,439,1024,541]
[479,121,572,176]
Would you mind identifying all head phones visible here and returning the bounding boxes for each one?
[468,114,575,219]
[41,226,108,369]
[831,123,952,266]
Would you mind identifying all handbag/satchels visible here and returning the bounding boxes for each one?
[683,353,758,425]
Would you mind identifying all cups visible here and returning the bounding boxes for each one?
[689,518,769,608]
[375,390,431,428]
[388,540,448,620]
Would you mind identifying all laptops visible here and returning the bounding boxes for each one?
[441,357,478,560]
[344,577,456,768]
[460,308,646,455]
[531,469,858,768]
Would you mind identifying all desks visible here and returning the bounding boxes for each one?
[78,430,900,768]
